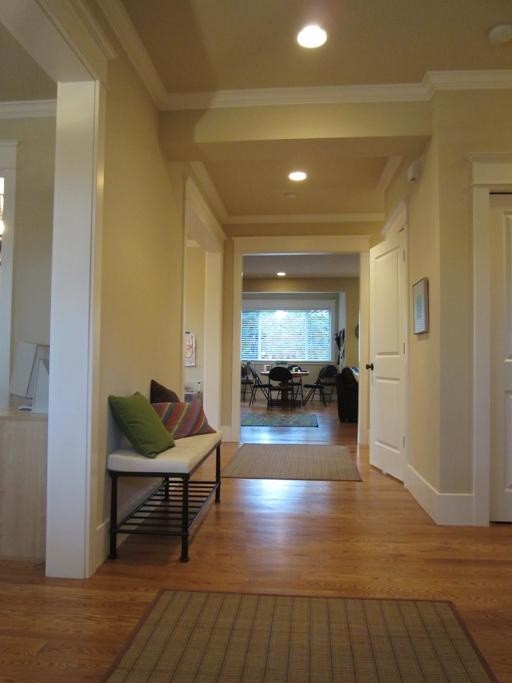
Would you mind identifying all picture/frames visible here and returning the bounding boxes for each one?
[411,275,428,335]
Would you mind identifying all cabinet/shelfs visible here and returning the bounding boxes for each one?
[107,430,222,563]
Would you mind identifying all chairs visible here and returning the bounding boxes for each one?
[241,361,357,422]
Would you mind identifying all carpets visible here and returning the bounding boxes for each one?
[221,411,362,480]
[100,586,499,683]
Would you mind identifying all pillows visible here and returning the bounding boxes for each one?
[107,378,217,457]
